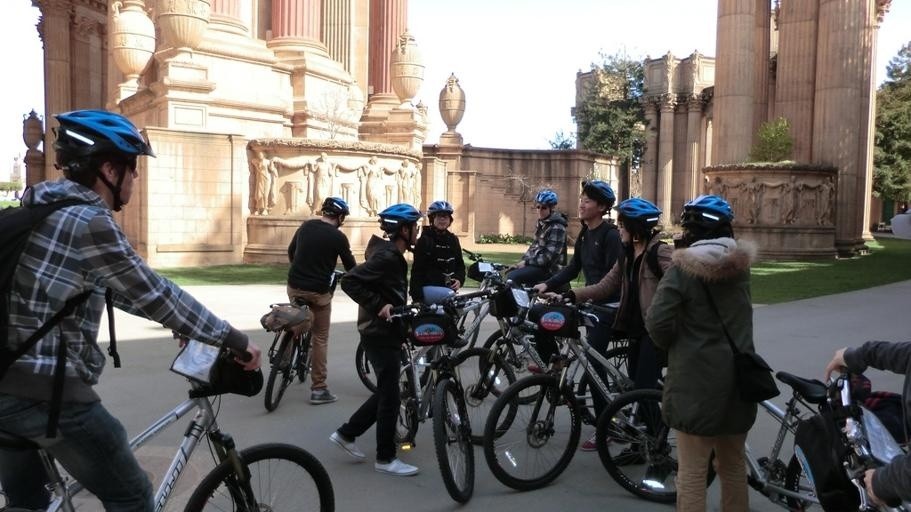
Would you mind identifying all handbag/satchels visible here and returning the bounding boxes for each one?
[260,303,314,334]
[735,342,780,404]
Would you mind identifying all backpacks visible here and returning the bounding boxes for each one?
[0,195,121,440]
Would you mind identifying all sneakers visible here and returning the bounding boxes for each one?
[374,457,419,477]
[580,430,613,452]
[328,431,369,462]
[309,389,339,405]
[452,334,468,346]
[610,447,648,466]
[528,363,544,374]
[638,460,674,490]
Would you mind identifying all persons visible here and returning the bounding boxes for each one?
[330,204,420,476]
[245,148,428,221]
[506,190,568,288]
[825,342,911,509]
[643,195,759,512]
[701,175,839,232]
[411,201,467,349]
[1,109,262,512]
[279,197,358,404]
[543,198,677,489]
[528,177,622,451]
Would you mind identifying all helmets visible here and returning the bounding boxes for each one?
[321,197,351,229]
[534,189,558,221]
[426,200,454,233]
[50,108,157,213]
[377,203,424,252]
[680,194,735,247]
[617,197,664,244]
[582,179,616,215]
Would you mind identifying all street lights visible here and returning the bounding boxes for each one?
[619,79,644,202]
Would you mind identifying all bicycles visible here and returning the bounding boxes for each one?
[0,326,338,512]
[264,269,347,412]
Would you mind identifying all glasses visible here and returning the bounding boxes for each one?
[432,214,451,218]
[536,206,550,210]
